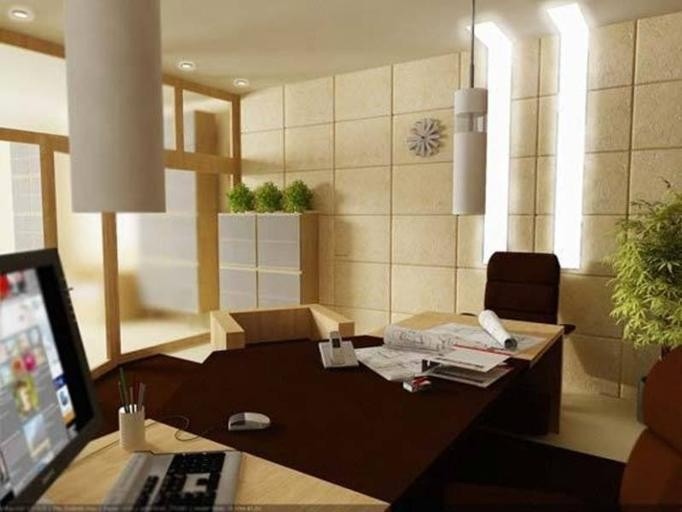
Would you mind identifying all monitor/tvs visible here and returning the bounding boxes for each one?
[0,247,107,512]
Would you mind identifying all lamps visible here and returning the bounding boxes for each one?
[61,1,167,215]
[451,1,491,217]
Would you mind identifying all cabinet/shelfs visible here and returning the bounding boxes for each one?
[217,210,319,310]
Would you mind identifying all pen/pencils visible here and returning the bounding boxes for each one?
[118,365,145,413]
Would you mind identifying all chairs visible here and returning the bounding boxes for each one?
[442,342,681,512]
[483,250,562,324]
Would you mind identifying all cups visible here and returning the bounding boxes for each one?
[117,403,147,448]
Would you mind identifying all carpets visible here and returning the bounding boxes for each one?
[387,427,625,510]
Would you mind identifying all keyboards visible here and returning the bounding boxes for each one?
[99,451,242,512]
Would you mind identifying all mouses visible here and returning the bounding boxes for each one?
[228,412,271,431]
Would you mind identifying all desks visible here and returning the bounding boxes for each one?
[32,313,577,512]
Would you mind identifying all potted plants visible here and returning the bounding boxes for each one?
[600,174,682,424]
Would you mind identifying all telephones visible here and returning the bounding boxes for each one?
[319,331,359,369]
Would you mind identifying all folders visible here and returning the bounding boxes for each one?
[420,344,515,389]
